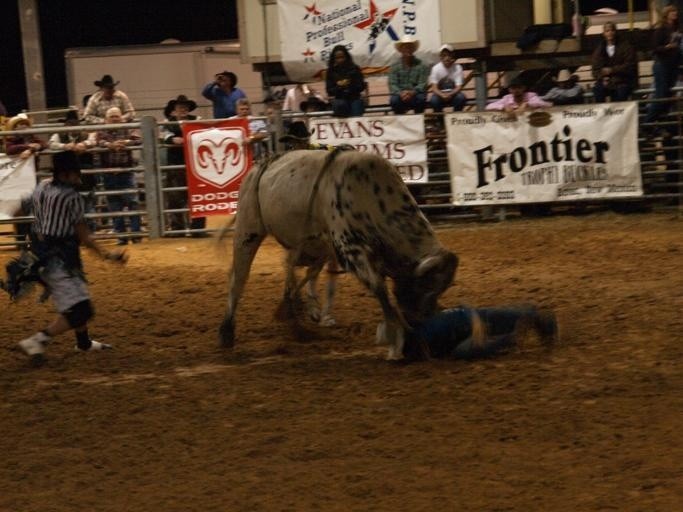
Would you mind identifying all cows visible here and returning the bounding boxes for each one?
[214,146,459,363]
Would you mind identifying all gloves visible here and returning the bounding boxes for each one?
[109,249,130,265]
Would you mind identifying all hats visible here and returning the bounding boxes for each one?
[302,97,328,111]
[440,44,454,53]
[506,78,527,88]
[8,112,32,129]
[395,35,420,52]
[216,72,237,87]
[280,120,315,142]
[95,76,120,87]
[552,69,578,84]
[168,95,197,112]
[58,109,86,123]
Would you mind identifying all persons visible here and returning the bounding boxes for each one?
[326,45,367,118]
[160,94,210,238]
[281,82,327,113]
[591,22,640,103]
[387,37,468,113]
[83,75,136,125]
[277,121,335,152]
[648,6,683,121]
[13,152,130,359]
[203,70,268,161]
[374,306,558,364]
[485,69,585,114]
[5,107,143,247]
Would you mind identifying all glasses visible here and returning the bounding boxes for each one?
[286,144,296,150]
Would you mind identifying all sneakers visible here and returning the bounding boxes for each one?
[74,340,113,353]
[18,338,48,366]
